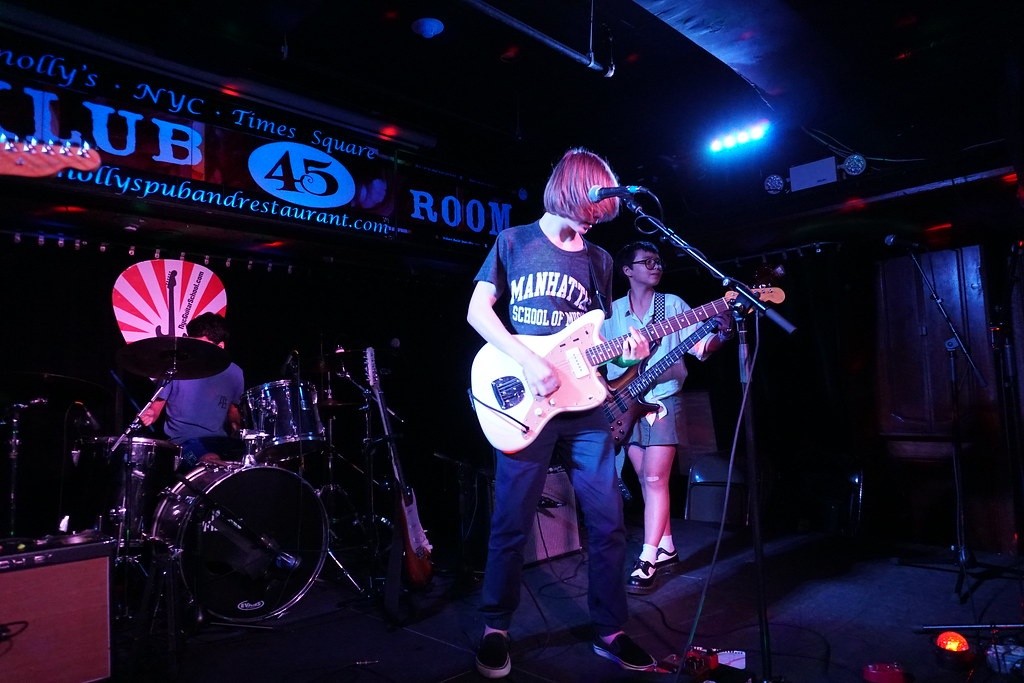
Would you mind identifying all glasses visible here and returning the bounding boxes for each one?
[632,258,663,272]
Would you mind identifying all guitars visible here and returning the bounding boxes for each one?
[601,307,753,448]
[471,284,786,454]
[363,346,434,587]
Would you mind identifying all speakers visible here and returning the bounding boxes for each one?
[0,528,118,683]
[521,464,582,570]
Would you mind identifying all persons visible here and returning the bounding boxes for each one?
[141,311,247,466]
[466,149,660,676]
[601,242,733,594]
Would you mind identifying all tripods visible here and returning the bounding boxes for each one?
[889,248,1024,609]
[313,366,397,574]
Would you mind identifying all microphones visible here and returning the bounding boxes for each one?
[589,184,650,203]
[83,405,102,431]
[281,350,297,379]
[537,500,563,507]
[885,235,920,249]
[281,554,304,571]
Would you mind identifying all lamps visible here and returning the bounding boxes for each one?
[764,154,867,195]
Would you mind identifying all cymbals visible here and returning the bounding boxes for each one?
[122,336,232,380]
[318,401,369,422]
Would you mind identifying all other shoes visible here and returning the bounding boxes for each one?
[476,629,511,678]
[593,633,657,670]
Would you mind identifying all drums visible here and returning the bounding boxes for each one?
[151,461,331,624]
[82,437,183,550]
[238,379,327,463]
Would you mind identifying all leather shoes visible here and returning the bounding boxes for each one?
[625,547,680,595]
